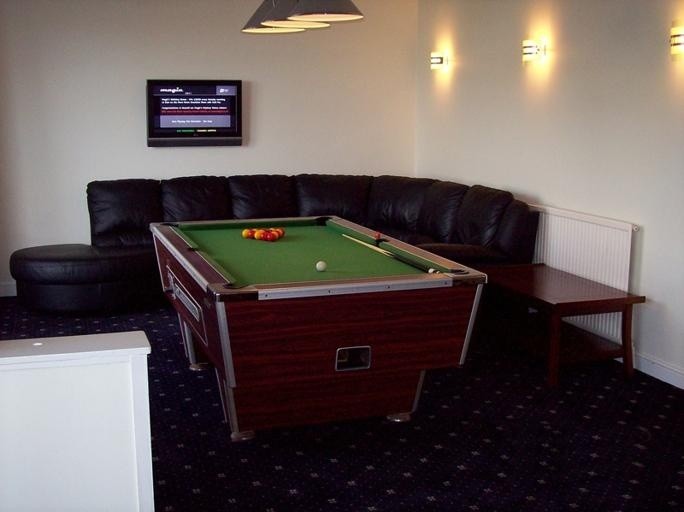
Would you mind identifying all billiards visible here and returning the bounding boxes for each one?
[242,227,284,240]
[316,261,327,271]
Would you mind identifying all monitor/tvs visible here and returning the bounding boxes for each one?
[147,79,242,146]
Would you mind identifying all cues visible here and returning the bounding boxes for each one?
[341,233,439,273]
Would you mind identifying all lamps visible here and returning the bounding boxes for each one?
[670,27,684,54]
[241,0,365,33]
[523,39,545,61]
[430,52,448,69]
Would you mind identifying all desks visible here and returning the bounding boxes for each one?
[149,214,489,443]
[464,263,645,390]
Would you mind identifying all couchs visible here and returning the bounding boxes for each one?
[88,174,539,319]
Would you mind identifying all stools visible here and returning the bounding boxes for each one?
[10,244,102,312]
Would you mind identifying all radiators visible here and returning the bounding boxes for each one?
[529,205,639,346]
